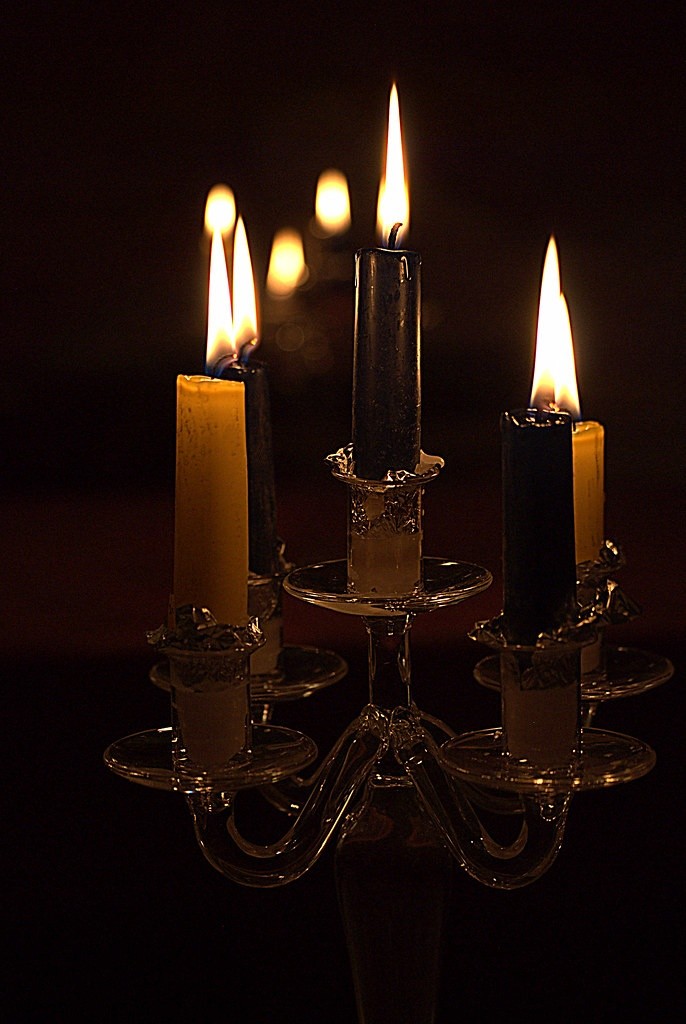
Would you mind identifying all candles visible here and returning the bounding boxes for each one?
[344,80,424,602]
[165,218,280,778]
[499,233,607,767]
